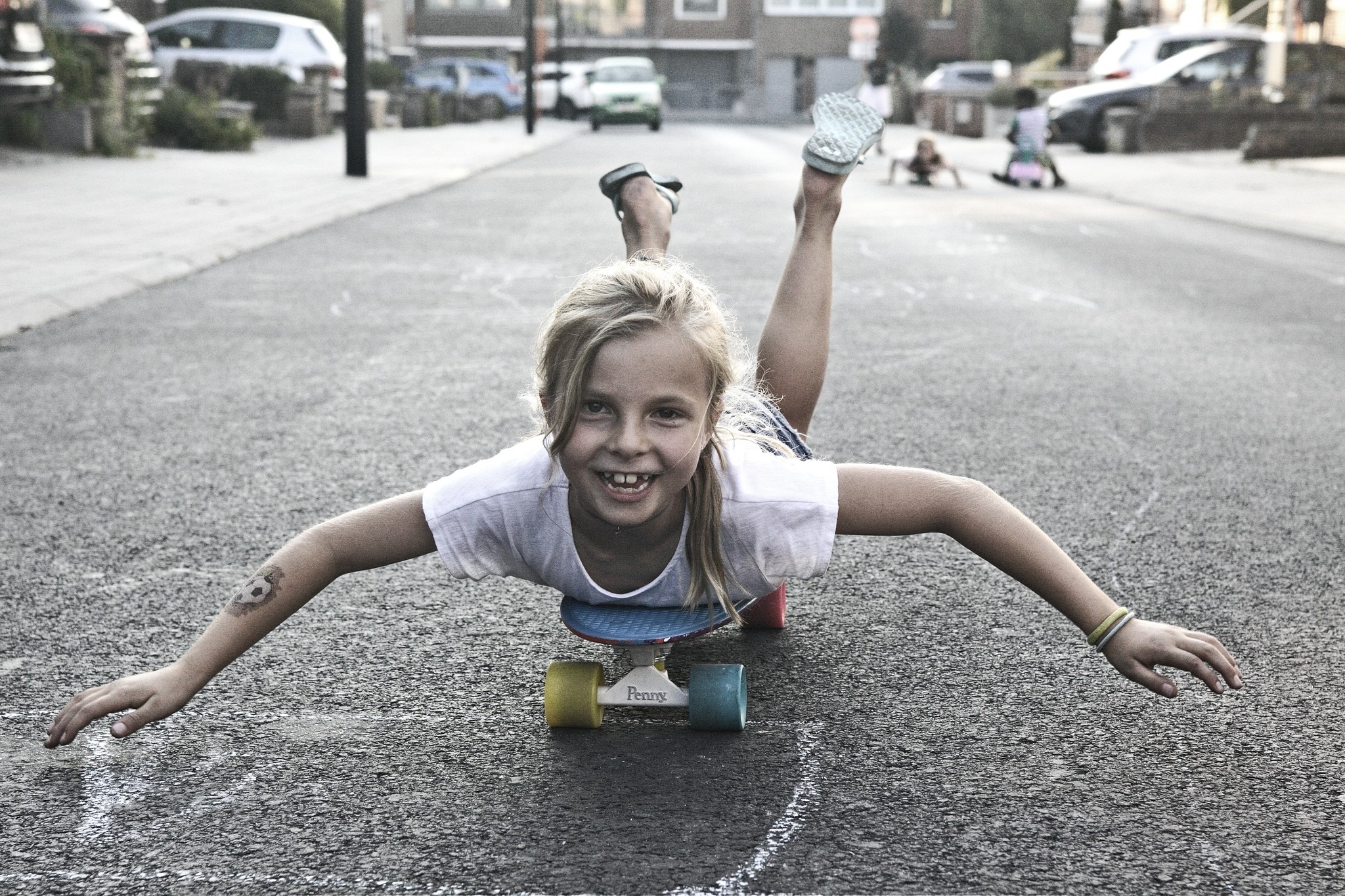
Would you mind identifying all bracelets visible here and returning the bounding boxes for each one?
[1096,611,1136,653]
[1087,606,1129,646]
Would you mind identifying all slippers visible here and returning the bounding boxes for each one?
[598,162,683,221]
[802,91,885,175]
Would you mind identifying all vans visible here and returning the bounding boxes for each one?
[921,56,1010,96]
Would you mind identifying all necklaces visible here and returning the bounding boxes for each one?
[575,494,672,535]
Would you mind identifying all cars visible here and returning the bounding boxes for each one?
[1049,38,1344,156]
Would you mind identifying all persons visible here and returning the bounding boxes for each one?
[40,94,1244,751]
[991,85,1066,189]
[880,139,965,188]
[856,44,902,156]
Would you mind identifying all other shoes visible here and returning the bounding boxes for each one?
[1054,177,1065,188]
[993,173,1007,183]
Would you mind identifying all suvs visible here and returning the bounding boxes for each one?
[514,59,597,120]
[141,1,350,121]
[591,54,667,131]
[407,55,523,118]
[1,0,164,154]
[1080,24,1279,86]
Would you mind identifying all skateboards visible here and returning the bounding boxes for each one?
[545,580,787,731]
